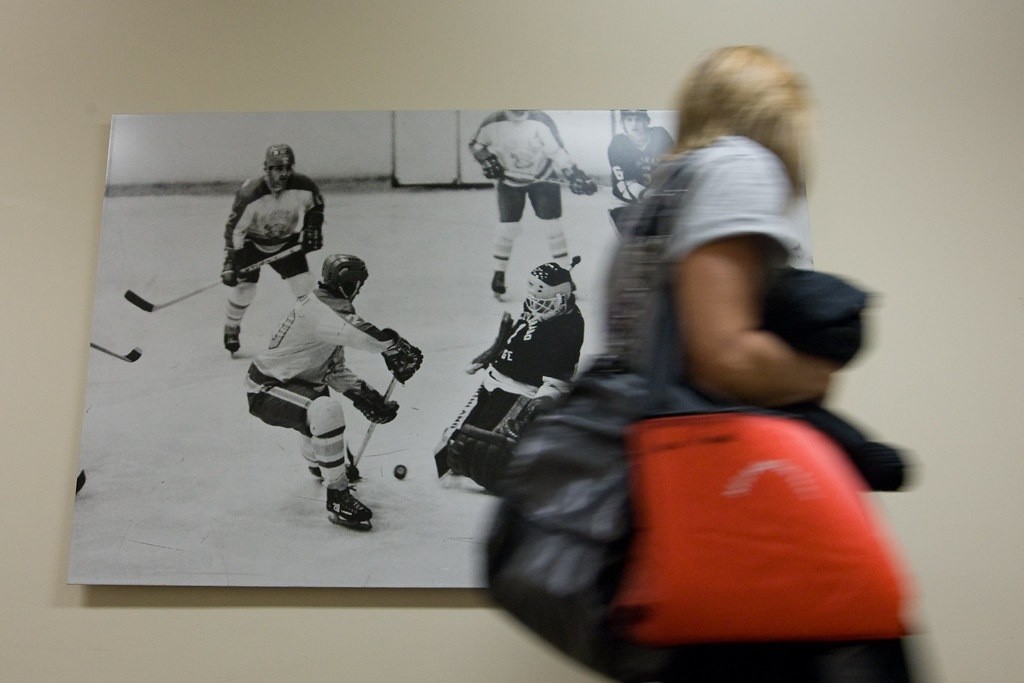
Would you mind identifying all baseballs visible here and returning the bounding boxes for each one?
[393,464,408,481]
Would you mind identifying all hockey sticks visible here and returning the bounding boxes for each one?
[505,168,613,198]
[353,375,396,469]
[91,341,148,365]
[125,245,302,315]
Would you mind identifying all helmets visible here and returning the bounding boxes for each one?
[264,144,295,170]
[621,110,648,120]
[525,262,577,319]
[322,253,369,302]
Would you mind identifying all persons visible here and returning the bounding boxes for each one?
[218,111,676,535]
[606,44,912,683]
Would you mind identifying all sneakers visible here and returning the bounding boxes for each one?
[225,334,240,357]
[491,270,506,302]
[326,488,372,531]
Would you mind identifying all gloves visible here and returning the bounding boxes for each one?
[221,257,238,287]
[297,229,323,252]
[343,379,399,424]
[561,164,597,195]
[381,336,424,383]
[473,148,504,179]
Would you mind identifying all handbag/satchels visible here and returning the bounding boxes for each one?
[606,409,909,647]
[485,354,716,683]
